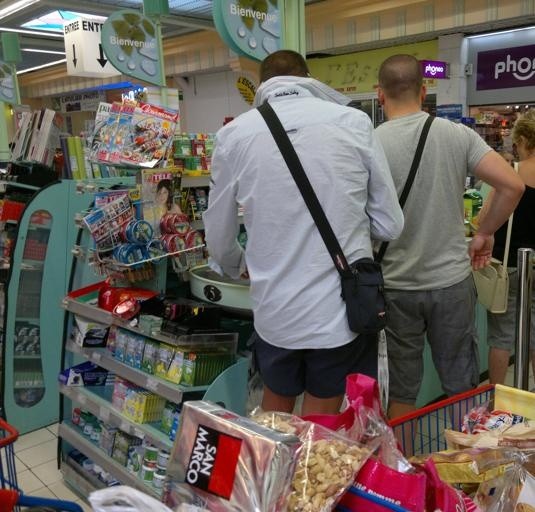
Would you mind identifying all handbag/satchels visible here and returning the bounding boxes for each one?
[471,256,511,314]
[338,255,389,336]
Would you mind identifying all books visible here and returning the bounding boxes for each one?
[10,98,184,251]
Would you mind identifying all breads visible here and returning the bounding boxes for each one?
[406,420,535,512]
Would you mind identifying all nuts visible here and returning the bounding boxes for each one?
[256,413,370,512]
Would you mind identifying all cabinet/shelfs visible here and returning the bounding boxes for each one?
[55,284,240,511]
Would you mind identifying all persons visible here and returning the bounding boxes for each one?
[202,50,407,416]
[485,107,535,411]
[372,54,524,456]
